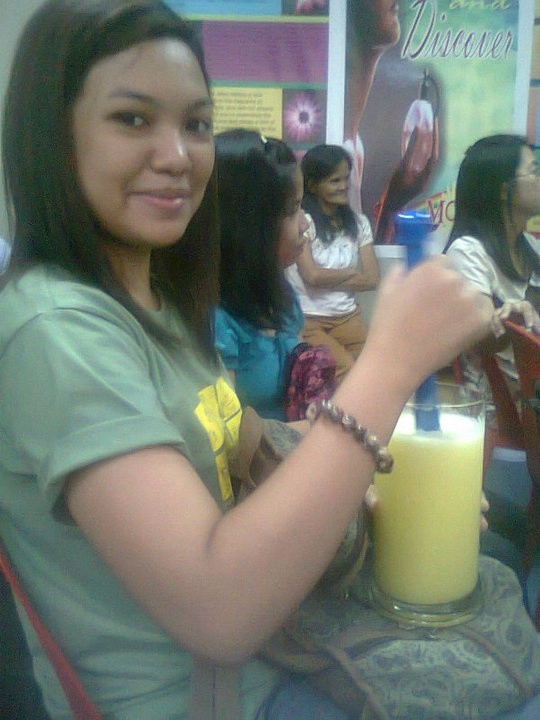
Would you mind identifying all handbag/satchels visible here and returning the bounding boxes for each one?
[284,341,335,421]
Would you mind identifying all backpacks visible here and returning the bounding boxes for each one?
[188,405,540,720]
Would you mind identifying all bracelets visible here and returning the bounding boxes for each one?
[316,398,396,474]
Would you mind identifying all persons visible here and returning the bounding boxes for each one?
[0,0,493,720]
[210,128,305,421]
[442,132,540,451]
[344,0,400,214]
[283,144,382,394]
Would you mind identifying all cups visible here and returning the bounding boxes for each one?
[367,381,485,628]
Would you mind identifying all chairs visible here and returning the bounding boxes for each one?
[0,548,105,720]
[450,319,540,571]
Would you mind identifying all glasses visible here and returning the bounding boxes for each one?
[512,167,540,176]
